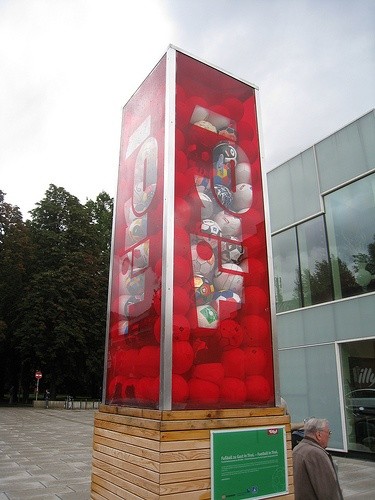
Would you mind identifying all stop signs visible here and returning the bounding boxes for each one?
[35,372,42,380]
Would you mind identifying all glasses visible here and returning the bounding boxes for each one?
[320,430,332,435]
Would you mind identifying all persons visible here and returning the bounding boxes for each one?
[45,388,50,408]
[292,418,344,500]
